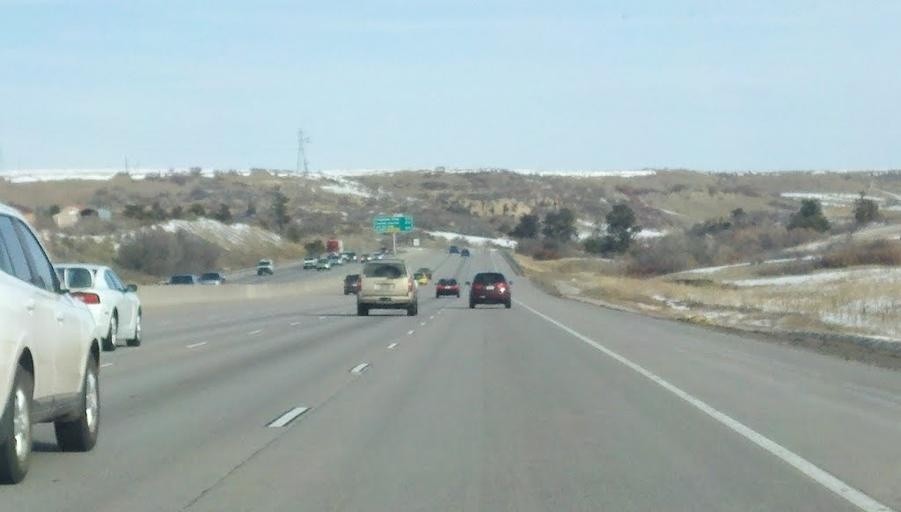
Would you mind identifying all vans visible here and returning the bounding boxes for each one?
[355,258,419,316]
[256,257,274,276]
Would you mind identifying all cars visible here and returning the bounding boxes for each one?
[448,245,470,258]
[167,271,226,287]
[0,202,104,488]
[465,272,517,309]
[301,239,433,296]
[48,261,145,350]
[434,278,461,298]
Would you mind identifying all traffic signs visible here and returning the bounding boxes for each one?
[372,215,415,234]
